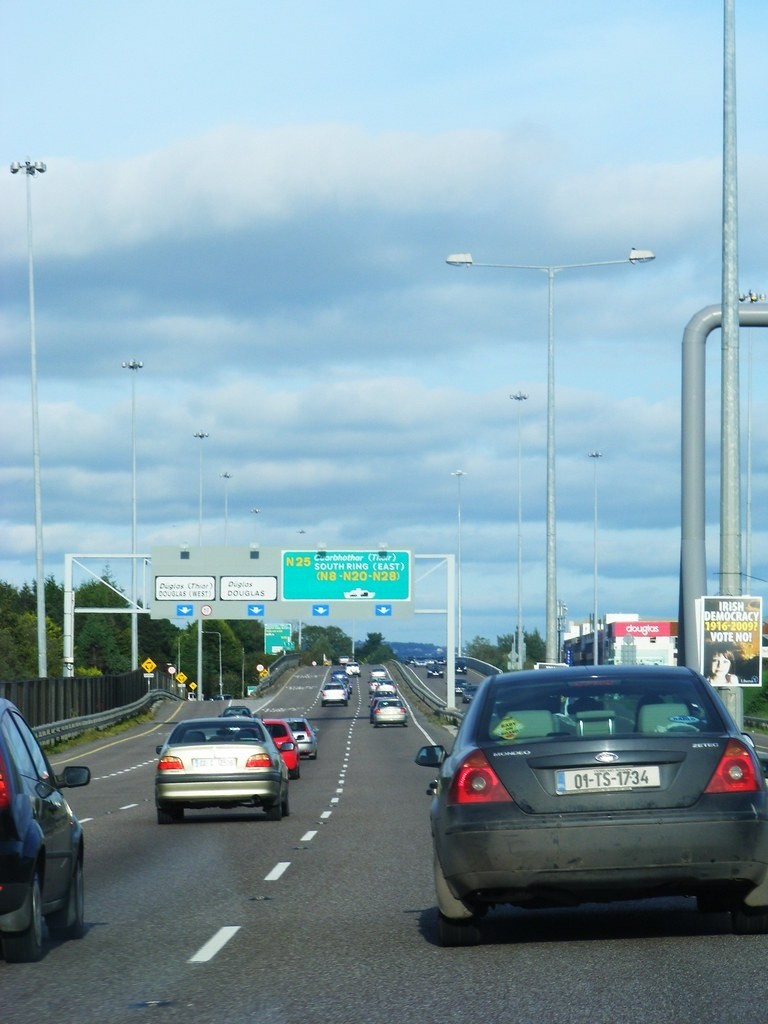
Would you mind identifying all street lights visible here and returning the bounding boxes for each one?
[588,451,604,667]
[220,471,234,549]
[451,470,468,658]
[178,634,190,673]
[509,391,530,672]
[447,249,655,674]
[738,290,767,596]
[121,358,145,671]
[194,431,209,701]
[9,160,48,679]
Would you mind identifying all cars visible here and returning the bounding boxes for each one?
[240,719,305,781]
[414,665,768,930]
[188,692,233,701]
[406,656,480,704]
[0,696,92,964]
[321,655,399,724]
[372,699,409,728]
[218,706,253,731]
[284,718,320,760]
[155,717,295,825]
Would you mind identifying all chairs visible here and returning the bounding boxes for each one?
[495,709,560,741]
[271,727,283,737]
[185,730,205,743]
[235,729,259,742]
[637,701,690,736]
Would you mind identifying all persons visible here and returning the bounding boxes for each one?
[705,646,739,687]
